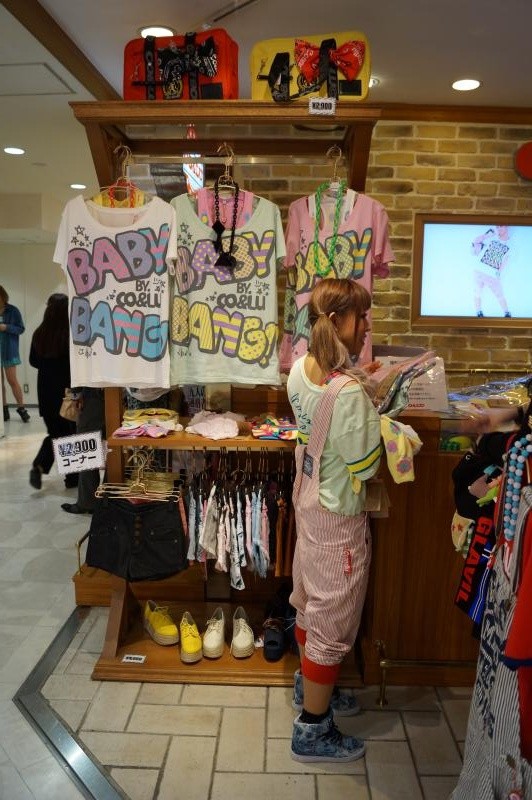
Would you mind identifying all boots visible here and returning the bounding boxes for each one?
[292,669,360,715]
[292,709,364,763]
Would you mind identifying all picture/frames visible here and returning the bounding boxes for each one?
[409,212,532,330]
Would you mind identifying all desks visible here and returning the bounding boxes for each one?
[355,405,521,689]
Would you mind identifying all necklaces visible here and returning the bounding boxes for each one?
[212,180,239,266]
[109,182,136,207]
[314,180,348,276]
[504,434,532,541]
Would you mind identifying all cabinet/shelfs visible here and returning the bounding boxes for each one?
[67,98,391,689]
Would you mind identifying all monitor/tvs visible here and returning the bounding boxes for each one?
[407,210,532,329]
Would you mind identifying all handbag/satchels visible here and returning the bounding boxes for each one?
[59,389,84,422]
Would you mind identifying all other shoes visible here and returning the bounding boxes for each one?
[3,405,8,419]
[478,311,484,318]
[17,407,30,419]
[29,467,42,489]
[504,312,511,318]
[60,501,93,513]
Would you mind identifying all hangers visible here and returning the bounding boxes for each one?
[194,144,261,209]
[87,145,154,201]
[90,445,295,503]
[321,145,348,193]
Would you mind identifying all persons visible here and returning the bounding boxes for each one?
[286,279,385,761]
[62,387,106,514]
[30,293,78,490]
[451,378,532,639]
[0,285,31,422]
[474,226,512,317]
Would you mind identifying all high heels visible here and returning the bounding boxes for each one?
[143,601,178,645]
[202,608,226,657]
[232,604,253,657]
[264,605,286,660]
[179,610,203,663]
